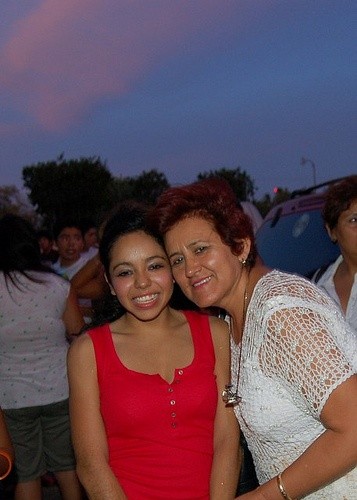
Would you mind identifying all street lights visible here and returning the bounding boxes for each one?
[299,157,317,186]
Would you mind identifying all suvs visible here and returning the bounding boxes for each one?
[254,172,356,278]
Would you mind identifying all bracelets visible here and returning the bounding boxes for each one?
[277,472,292,500]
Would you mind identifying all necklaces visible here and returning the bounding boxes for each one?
[222,271,250,407]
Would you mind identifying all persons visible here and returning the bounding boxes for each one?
[0,173,357,500]
